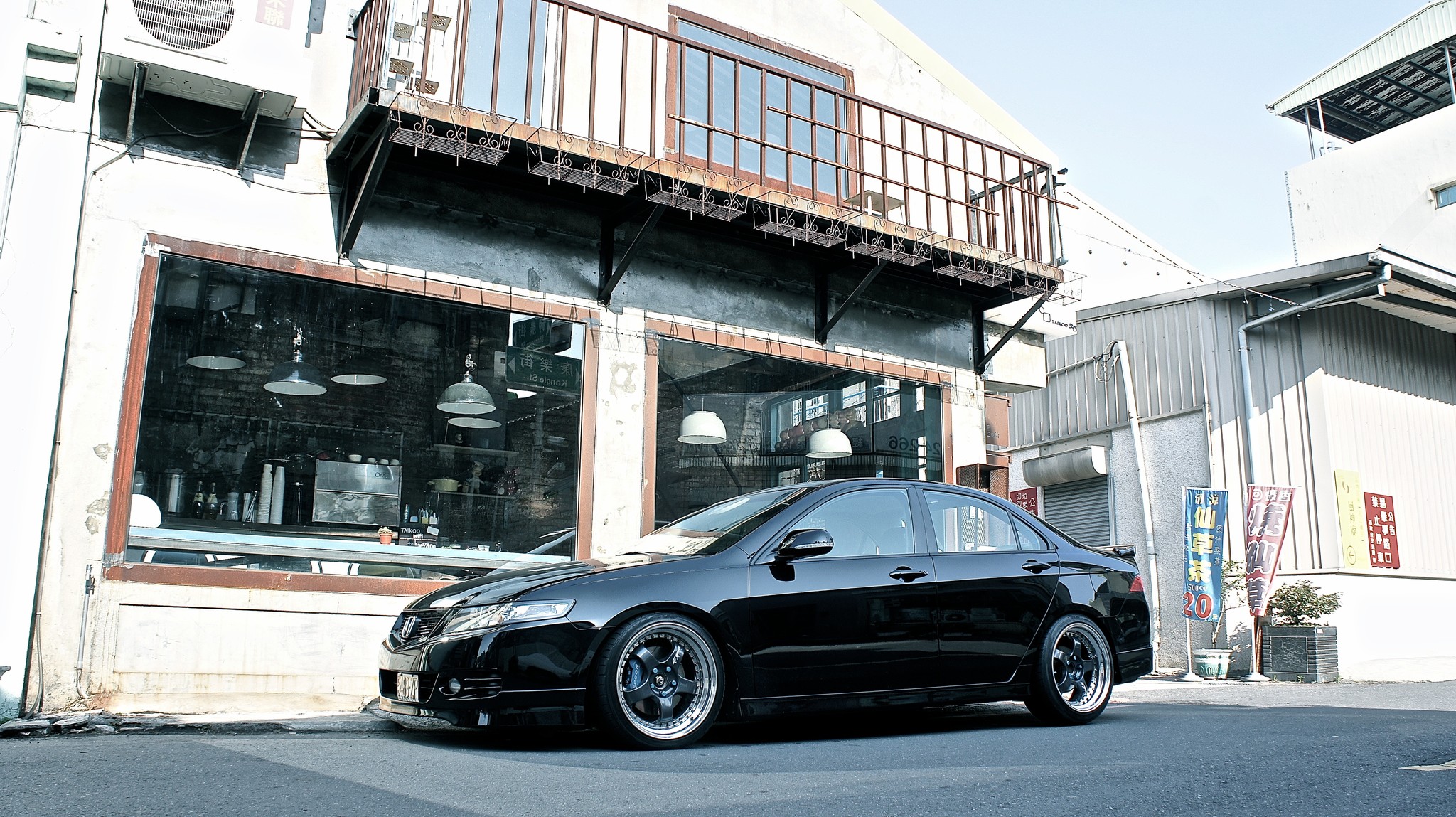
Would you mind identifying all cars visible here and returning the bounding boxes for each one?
[489,522,671,577]
[382,481,1153,752]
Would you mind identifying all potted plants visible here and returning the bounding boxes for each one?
[1262,579,1343,683]
[1194,558,1247,681]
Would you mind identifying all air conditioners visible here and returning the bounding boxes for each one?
[99,0,312,120]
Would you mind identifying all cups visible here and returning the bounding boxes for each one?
[256,464,285,524]
[219,491,257,522]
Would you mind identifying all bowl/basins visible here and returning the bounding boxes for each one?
[366,458,376,465]
[379,459,389,465]
[348,454,362,463]
[390,459,400,466]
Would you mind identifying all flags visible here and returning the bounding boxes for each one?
[1246,483,1296,617]
[1181,487,1228,622]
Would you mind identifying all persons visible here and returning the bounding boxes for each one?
[466,466,485,494]
[313,452,336,477]
[455,432,463,443]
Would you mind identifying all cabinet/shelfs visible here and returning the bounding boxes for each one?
[141,407,515,541]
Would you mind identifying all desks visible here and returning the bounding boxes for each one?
[844,190,905,225]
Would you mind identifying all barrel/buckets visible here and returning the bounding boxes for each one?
[155,473,186,518]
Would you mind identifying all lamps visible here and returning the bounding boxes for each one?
[264,313,327,396]
[448,337,503,429]
[804,369,852,459]
[678,363,727,444]
[330,318,388,385]
[437,371,496,414]
[186,335,247,370]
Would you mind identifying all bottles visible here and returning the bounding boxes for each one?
[191,480,204,520]
[206,482,218,520]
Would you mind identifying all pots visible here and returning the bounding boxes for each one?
[427,476,463,492]
